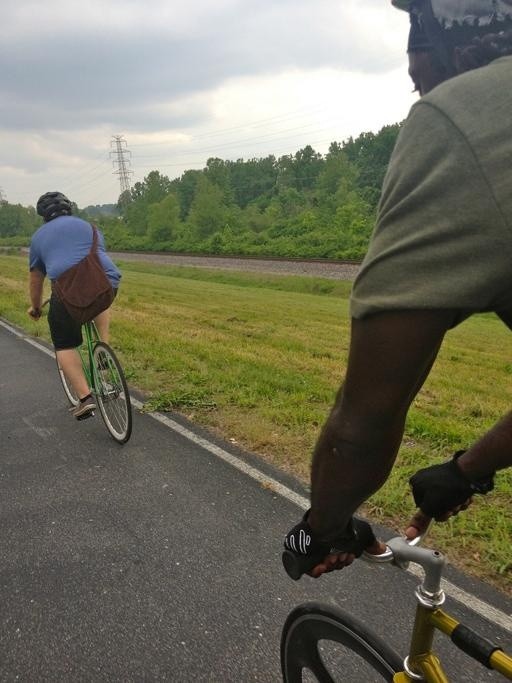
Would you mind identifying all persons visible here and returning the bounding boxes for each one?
[27,190,122,420]
[281,1,511,579]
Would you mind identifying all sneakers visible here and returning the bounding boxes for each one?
[102,382,121,396]
[72,396,97,417]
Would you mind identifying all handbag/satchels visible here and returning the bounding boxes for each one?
[53,253,115,326]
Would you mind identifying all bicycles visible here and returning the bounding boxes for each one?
[33,295,132,444]
[280,485,512,682]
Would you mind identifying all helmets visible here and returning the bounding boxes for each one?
[392,2,510,72]
[36,192,71,222]
